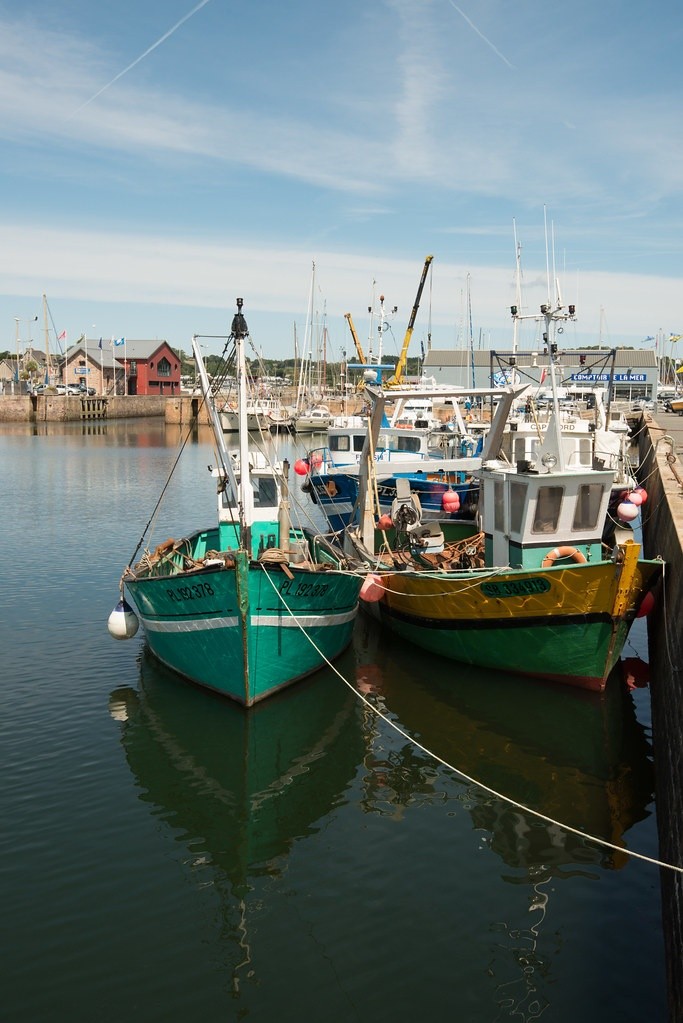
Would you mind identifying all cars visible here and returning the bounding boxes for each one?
[69,383,96,396]
[54,384,82,396]
[27,383,48,396]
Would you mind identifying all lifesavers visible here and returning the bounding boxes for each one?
[539,545,588,569]
[396,424,414,429]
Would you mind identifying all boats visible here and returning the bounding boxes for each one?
[118,297,364,709]
[365,396,672,694]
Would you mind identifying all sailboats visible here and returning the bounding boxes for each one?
[294,201,645,556]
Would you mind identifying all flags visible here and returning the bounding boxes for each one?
[77,334,84,344]
[109,338,114,346]
[58,331,65,341]
[98,337,102,349]
[114,338,124,346]
[668,332,681,341]
[541,368,547,382]
